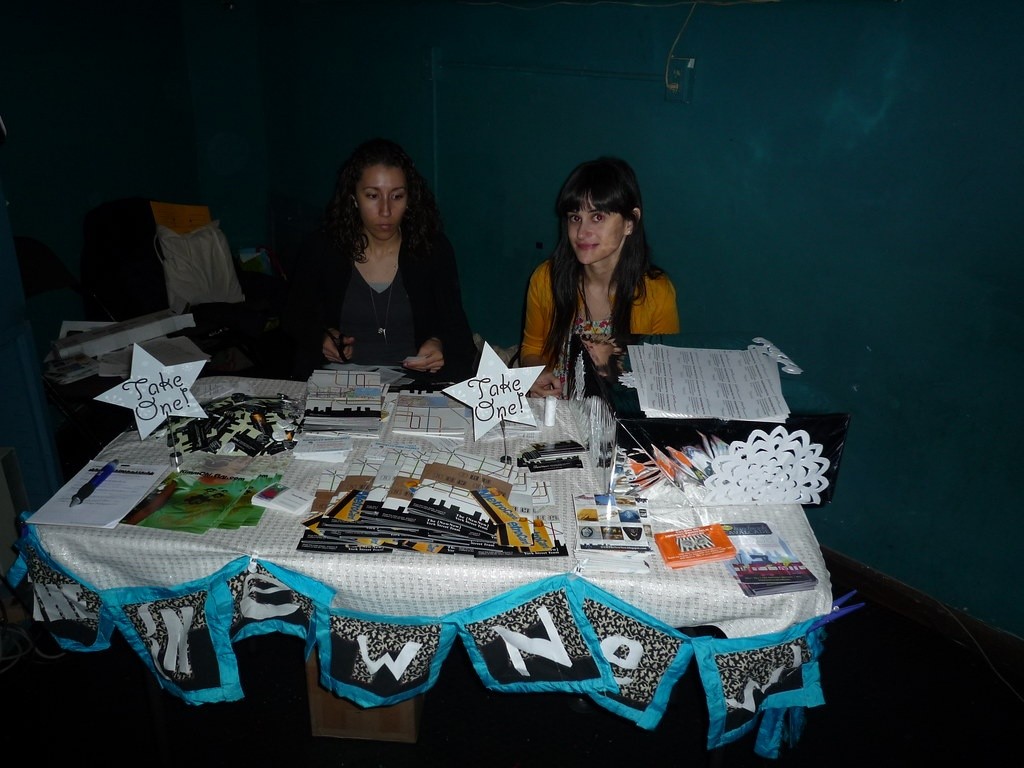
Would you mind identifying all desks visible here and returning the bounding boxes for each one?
[25,373,832,642]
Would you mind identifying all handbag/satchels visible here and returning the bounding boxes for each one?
[152,219,246,307]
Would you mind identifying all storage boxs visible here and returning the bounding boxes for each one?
[302,643,425,742]
[50,297,197,360]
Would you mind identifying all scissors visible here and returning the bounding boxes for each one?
[321,324,350,361]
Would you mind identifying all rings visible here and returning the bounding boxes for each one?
[426,369,431,373]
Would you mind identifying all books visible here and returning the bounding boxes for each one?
[253,370,819,597]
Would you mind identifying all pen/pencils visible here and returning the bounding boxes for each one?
[68,457,120,509]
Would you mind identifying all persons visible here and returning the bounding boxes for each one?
[260,142,483,392]
[518,155,680,399]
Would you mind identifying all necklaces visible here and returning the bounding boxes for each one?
[363,264,400,340]
[580,290,613,339]
